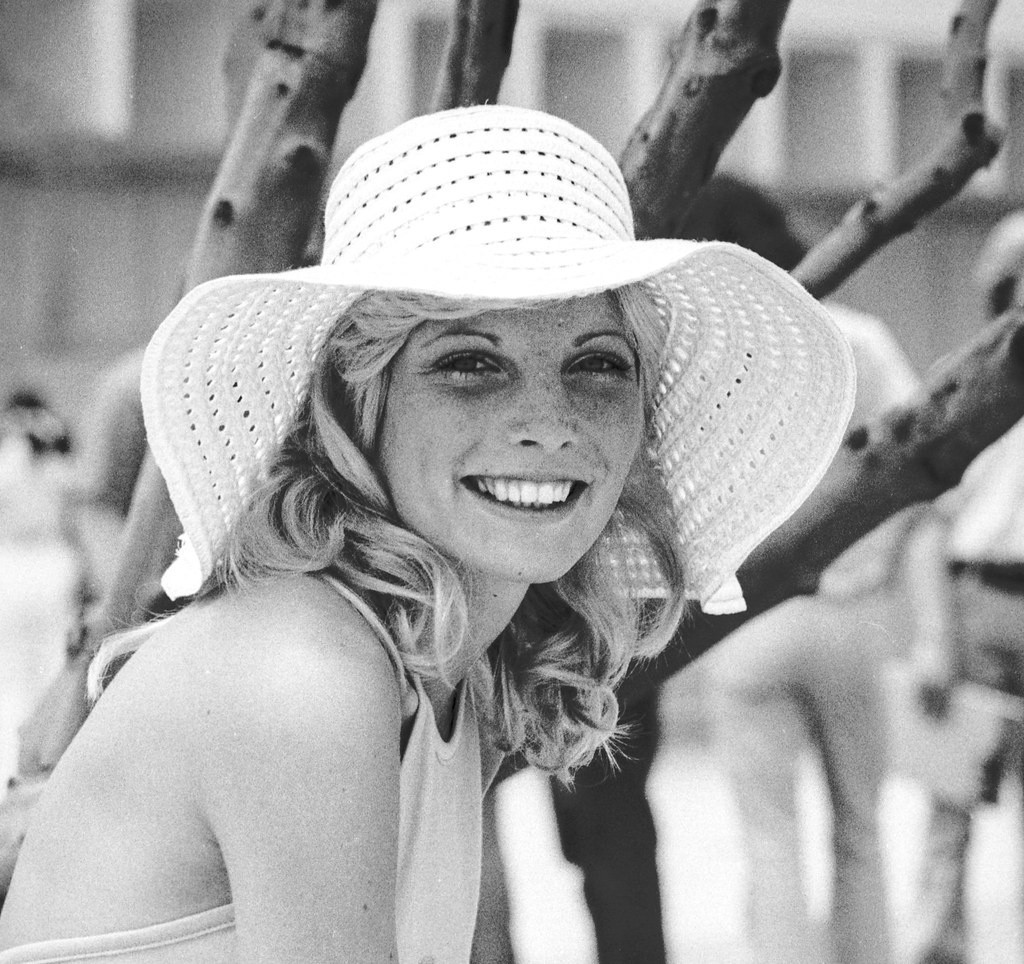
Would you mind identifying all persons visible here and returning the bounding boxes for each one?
[669,165,1021,964]
[1,102,691,963]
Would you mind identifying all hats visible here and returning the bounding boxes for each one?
[140,104,859,615]
[973,209,1024,294]
